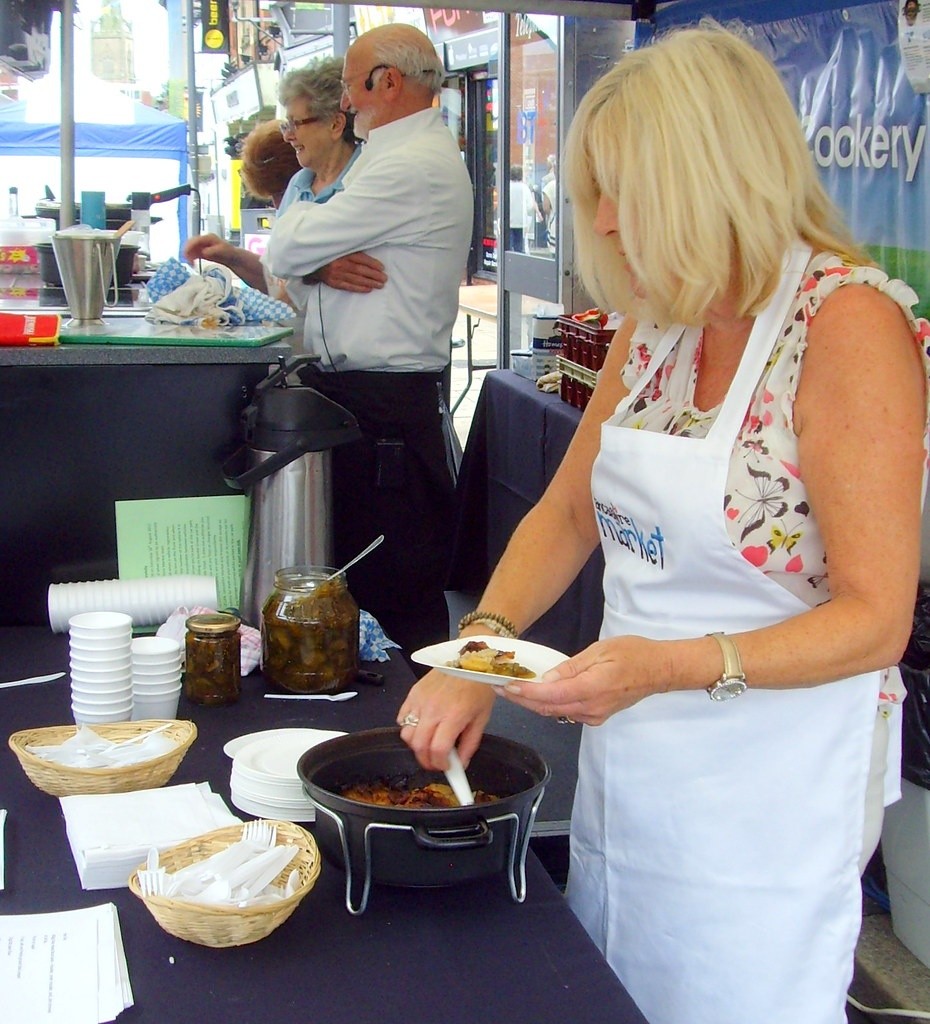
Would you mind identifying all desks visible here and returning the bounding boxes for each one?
[451,284,553,414]
[0,625,653,1024]
[444,369,930,968]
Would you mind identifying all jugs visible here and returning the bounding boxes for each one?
[219,353,363,630]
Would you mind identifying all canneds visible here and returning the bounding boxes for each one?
[259,566,360,696]
[185,613,243,707]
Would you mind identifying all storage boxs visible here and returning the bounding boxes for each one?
[556,315,617,412]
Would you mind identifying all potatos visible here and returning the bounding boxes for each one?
[425,783,503,806]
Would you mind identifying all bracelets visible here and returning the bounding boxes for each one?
[457,611,517,639]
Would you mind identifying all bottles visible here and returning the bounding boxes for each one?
[262,567,362,692]
[8,187,18,216]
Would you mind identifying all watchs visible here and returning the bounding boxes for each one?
[705,631,746,702]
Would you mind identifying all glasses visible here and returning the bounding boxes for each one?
[340,68,405,96]
[278,116,318,135]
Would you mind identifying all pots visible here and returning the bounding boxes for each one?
[35,184,191,227]
[36,241,140,285]
[296,726,552,886]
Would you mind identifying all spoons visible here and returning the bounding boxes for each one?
[264,692,359,701]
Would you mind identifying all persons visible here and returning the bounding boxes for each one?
[239,118,303,209]
[543,160,556,259]
[267,23,476,677]
[184,55,363,383]
[540,154,557,201]
[396,27,930,1024]
[509,165,543,254]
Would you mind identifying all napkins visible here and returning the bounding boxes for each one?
[58,781,243,891]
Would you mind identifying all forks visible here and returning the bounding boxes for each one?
[136,818,299,897]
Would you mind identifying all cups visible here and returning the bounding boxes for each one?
[48,574,217,725]
[52,230,120,318]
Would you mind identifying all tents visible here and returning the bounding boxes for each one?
[0,59,187,266]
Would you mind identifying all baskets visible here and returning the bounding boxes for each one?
[128,817,316,941]
[8,720,197,800]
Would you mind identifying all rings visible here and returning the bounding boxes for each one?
[404,715,419,727]
[556,716,575,725]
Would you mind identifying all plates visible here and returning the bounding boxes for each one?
[224,728,347,822]
[410,635,570,684]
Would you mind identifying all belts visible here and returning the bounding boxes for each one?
[318,371,441,390]
